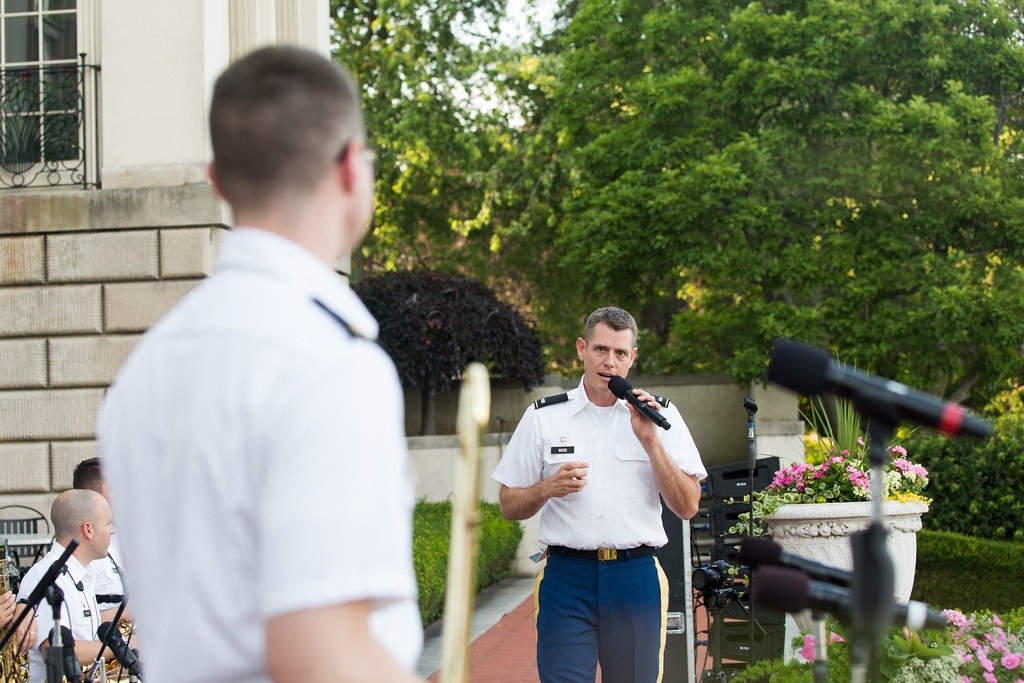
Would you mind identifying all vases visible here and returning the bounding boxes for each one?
[767,502,929,607]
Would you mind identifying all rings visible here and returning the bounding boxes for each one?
[30,631,35,634]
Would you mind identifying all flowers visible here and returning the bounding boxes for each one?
[719,350,934,540]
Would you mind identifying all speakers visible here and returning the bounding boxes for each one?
[705,456,781,599]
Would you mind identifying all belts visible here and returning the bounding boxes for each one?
[548,546,654,561]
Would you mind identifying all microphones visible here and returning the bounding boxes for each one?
[739,536,852,586]
[767,344,993,440]
[608,375,671,431]
[752,565,947,631]
[49,625,82,683]
[99,622,143,682]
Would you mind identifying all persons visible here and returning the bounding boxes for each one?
[96,48,432,683]
[491,306,708,683]
[0,458,139,683]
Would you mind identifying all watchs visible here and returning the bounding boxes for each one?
[122,637,132,651]
[16,598,36,612]
